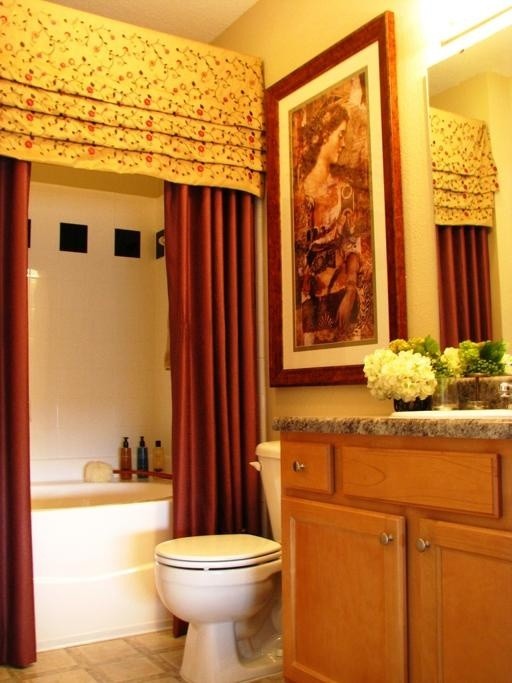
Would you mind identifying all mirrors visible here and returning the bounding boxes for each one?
[425,25,512,381]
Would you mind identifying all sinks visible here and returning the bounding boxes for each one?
[391,408,512,419]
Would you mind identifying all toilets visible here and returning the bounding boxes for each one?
[155,440,281,683]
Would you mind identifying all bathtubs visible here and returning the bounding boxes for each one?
[31,475,174,651]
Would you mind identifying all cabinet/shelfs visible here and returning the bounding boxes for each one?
[281,429,510,683]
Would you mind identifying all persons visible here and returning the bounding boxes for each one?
[294,102,374,345]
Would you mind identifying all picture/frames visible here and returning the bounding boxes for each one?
[262,10,407,386]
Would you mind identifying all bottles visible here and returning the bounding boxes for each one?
[152,440,165,472]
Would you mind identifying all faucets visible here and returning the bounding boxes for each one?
[499,382,512,397]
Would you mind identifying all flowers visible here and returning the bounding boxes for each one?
[442,340,512,376]
[362,334,447,403]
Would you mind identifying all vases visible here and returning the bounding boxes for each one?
[393,396,432,412]
